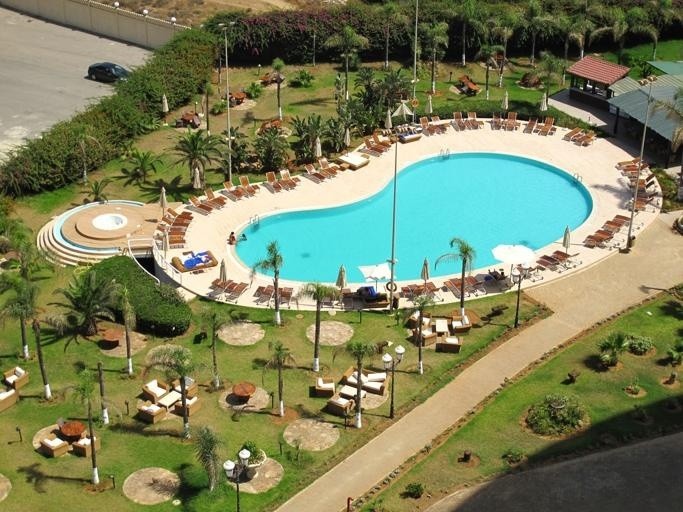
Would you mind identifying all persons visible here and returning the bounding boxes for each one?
[228,231,235,245]
[239,233,248,241]
[488,269,508,280]
[342,287,370,300]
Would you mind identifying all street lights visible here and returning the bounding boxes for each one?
[408,78,420,122]
[625,71,658,258]
[217,20,236,184]
[510,261,531,330]
[381,344,405,419]
[222,448,250,512]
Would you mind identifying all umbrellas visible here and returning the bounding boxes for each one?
[383,106,393,136]
[161,229,168,259]
[491,240,535,283]
[561,227,572,257]
[539,93,548,125]
[335,265,346,301]
[162,93,170,123]
[342,125,353,155]
[500,90,508,120]
[158,188,168,217]
[358,263,396,292]
[419,258,430,294]
[192,167,201,195]
[424,95,433,121]
[218,259,226,291]
[313,137,322,162]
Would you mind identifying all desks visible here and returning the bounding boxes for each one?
[233,380,256,400]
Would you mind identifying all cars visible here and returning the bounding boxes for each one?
[87,61,133,86]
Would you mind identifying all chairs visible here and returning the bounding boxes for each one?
[582,156,660,250]
[137,376,201,424]
[175,110,203,129]
[364,111,598,154]
[410,309,479,353]
[222,87,249,108]
[312,365,392,416]
[0,366,29,413]
[153,156,337,251]
[42,418,100,459]
